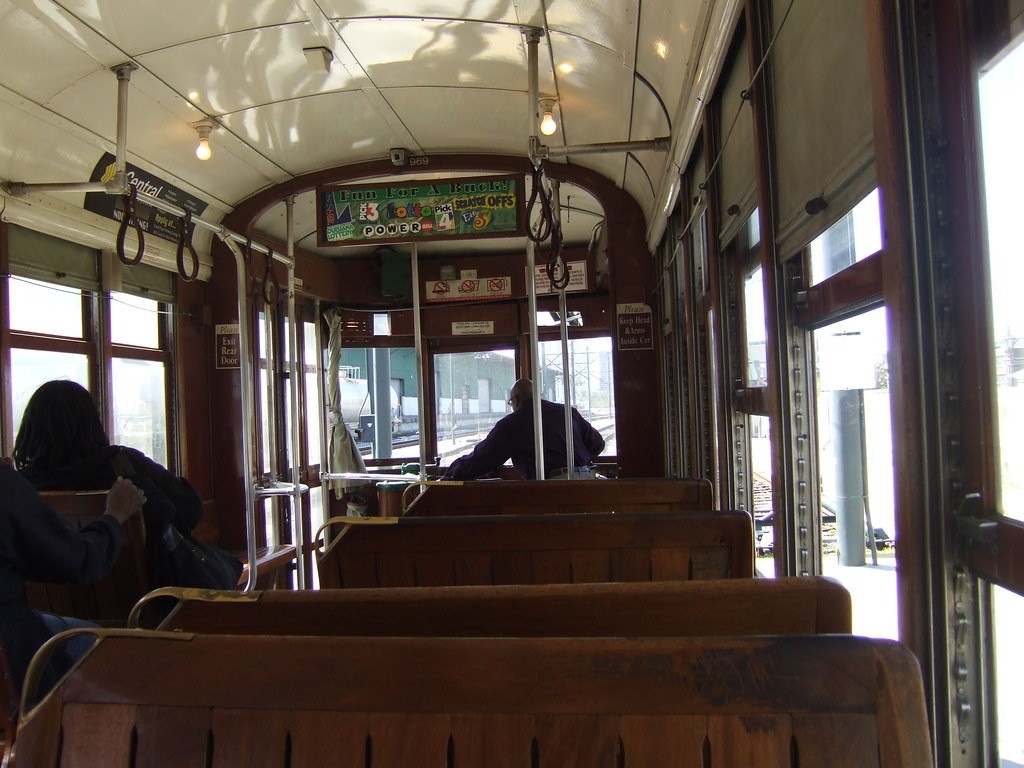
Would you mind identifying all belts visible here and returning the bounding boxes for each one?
[545,467,590,479]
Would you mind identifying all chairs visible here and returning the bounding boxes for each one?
[26,489,149,628]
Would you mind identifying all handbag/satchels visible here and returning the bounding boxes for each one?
[111,447,244,590]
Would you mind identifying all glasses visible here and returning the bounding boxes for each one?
[508,396,518,405]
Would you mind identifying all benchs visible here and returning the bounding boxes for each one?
[401,477,714,517]
[315,508,757,588]
[129,575,851,640]
[2,628,936,768]
[221,543,296,586]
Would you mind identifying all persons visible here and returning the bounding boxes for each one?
[12,380,244,623]
[439,378,606,480]
[0,455,149,702]
